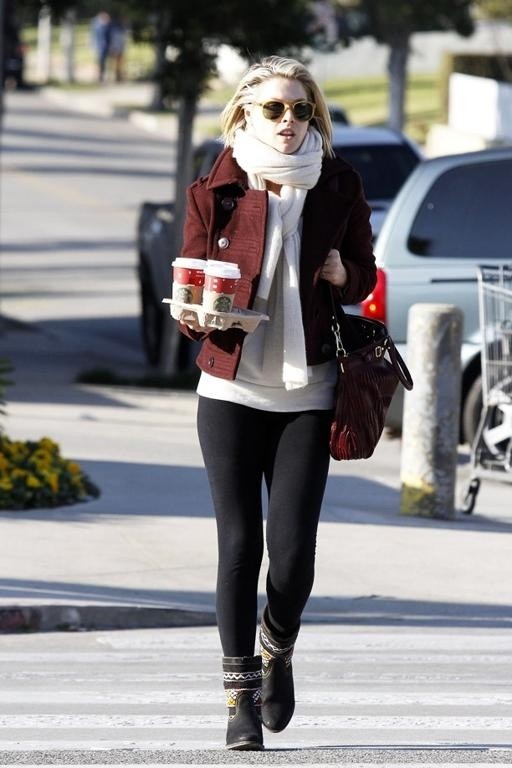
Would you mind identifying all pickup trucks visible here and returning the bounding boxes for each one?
[134,123,423,372]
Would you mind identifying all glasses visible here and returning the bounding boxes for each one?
[252,98,317,123]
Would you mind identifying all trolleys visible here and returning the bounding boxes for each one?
[462,264,512,516]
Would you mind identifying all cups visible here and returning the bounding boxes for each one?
[172,256,241,313]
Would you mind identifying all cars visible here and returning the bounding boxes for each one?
[355,148,512,471]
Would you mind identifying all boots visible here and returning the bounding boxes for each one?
[223,656,264,750]
[259,602,304,733]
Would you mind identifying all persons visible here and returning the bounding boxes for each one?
[175,55,377,749]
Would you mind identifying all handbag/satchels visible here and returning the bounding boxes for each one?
[329,314,413,462]
